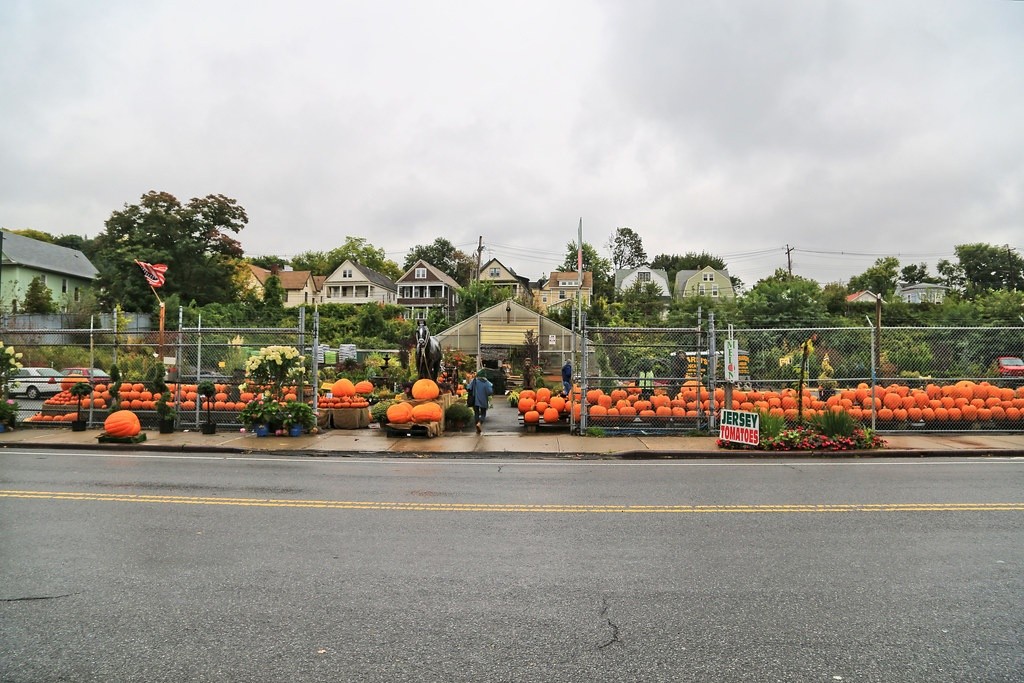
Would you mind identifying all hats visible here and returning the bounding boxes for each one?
[478,368,485,377]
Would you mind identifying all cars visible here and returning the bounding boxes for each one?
[57,368,112,384]
[188,370,226,384]
[163,356,198,380]
[992,357,1024,377]
[1,367,67,400]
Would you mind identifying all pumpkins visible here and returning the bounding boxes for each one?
[35,375,1023,440]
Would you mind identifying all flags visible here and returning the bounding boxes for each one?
[138,261,168,288]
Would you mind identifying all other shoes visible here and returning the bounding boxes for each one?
[476,424,482,432]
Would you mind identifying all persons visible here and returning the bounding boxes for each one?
[562,359,572,396]
[464,368,493,434]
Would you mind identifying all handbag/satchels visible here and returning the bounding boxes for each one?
[467,389,475,407]
